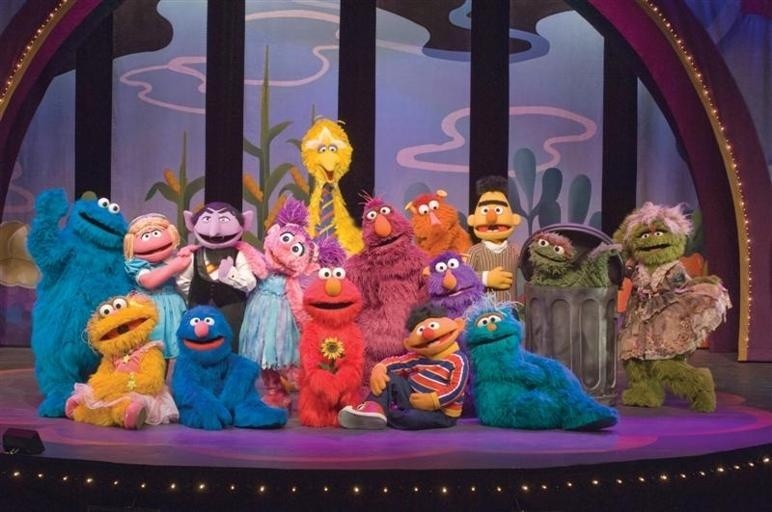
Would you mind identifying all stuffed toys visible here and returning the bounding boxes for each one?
[22,116,731,431]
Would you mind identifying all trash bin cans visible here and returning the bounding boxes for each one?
[515,222,626,407]
